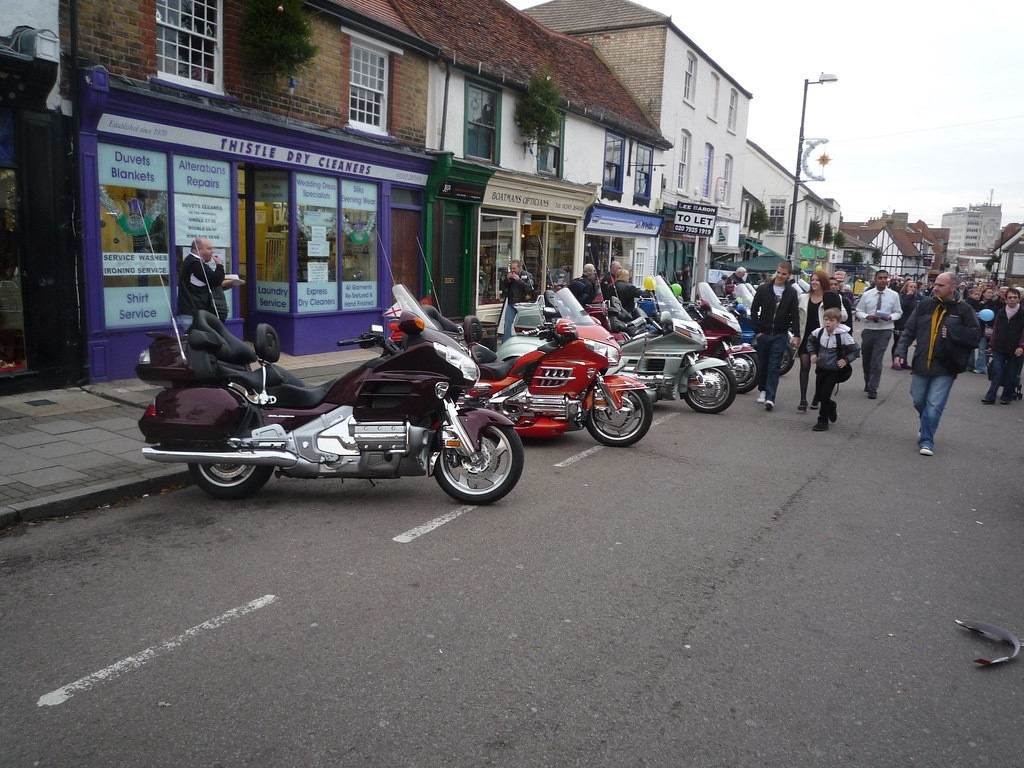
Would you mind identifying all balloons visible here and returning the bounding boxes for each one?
[815,266,823,271]
[800,261,807,269]
[644,277,656,290]
[978,309,994,321]
[672,284,682,297]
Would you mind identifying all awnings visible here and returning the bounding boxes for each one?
[745,240,773,254]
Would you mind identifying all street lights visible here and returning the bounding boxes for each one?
[787,72,838,262]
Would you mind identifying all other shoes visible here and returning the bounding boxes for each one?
[981,395,997,405]
[1001,398,1009,405]
[812,401,837,431]
[968,366,985,374]
[891,360,912,371]
[864,383,877,400]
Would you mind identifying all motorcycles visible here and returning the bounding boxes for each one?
[373,230,654,449]
[717,281,796,377]
[586,262,758,397]
[491,233,737,415]
[136,202,524,506]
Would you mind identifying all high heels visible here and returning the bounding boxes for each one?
[798,399,808,412]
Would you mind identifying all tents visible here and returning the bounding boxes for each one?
[720,252,803,275]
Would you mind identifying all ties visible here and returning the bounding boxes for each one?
[873,292,883,323]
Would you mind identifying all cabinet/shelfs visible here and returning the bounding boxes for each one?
[330,222,375,256]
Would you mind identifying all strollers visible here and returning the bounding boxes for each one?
[986,346,1023,400]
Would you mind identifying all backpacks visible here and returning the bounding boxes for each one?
[818,327,852,383]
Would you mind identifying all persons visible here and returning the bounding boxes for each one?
[893,272,982,456]
[682,263,693,301]
[856,270,903,400]
[714,267,746,297]
[865,273,1024,375]
[177,238,236,332]
[981,286,1024,405]
[807,308,861,431]
[568,261,642,313]
[789,270,855,412]
[501,260,535,344]
[750,262,802,411]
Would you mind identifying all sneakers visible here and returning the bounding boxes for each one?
[810,401,819,410]
[765,401,774,410]
[757,391,765,402]
[917,428,934,457]
